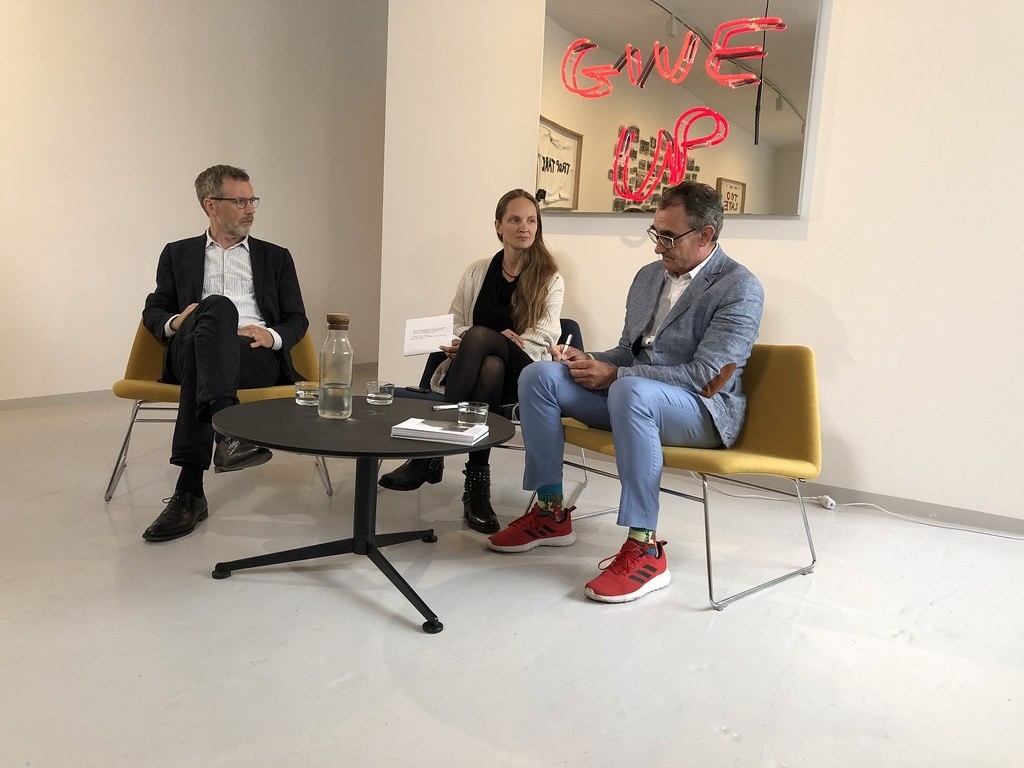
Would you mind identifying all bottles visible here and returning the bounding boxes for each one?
[318,313,354,419]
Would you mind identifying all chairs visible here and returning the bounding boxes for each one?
[524,344,822,609]
[378,318,589,481]
[102,312,333,502]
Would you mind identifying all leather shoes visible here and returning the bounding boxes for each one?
[377,456,445,491]
[213,437,273,473]
[142,489,208,541]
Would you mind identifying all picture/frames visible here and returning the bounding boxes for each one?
[535,0,831,218]
[538,115,583,210]
[715,177,746,214]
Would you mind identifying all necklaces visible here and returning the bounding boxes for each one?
[503,266,517,279]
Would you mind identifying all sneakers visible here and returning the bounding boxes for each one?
[585,541,670,601]
[486,502,577,553]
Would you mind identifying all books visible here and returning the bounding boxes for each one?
[391,417,489,447]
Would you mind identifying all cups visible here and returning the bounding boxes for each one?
[294,381,319,406]
[366,381,395,405]
[457,401,489,426]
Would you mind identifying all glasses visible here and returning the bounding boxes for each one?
[208,197,260,209]
[646,224,705,249]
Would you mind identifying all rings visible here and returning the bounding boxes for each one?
[509,334,515,339]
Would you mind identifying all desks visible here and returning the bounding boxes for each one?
[212,395,516,632]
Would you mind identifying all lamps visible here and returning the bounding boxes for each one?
[665,13,678,38]
[801,123,806,133]
[729,63,740,76]
[776,95,783,111]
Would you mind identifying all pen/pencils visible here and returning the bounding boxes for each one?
[562,334,572,356]
[432,404,458,410]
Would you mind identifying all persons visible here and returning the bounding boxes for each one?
[141,165,310,542]
[377,189,566,534]
[486,181,764,603]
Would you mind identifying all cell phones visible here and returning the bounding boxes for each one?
[406,386,430,394]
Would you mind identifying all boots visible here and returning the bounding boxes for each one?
[460,460,501,536]
